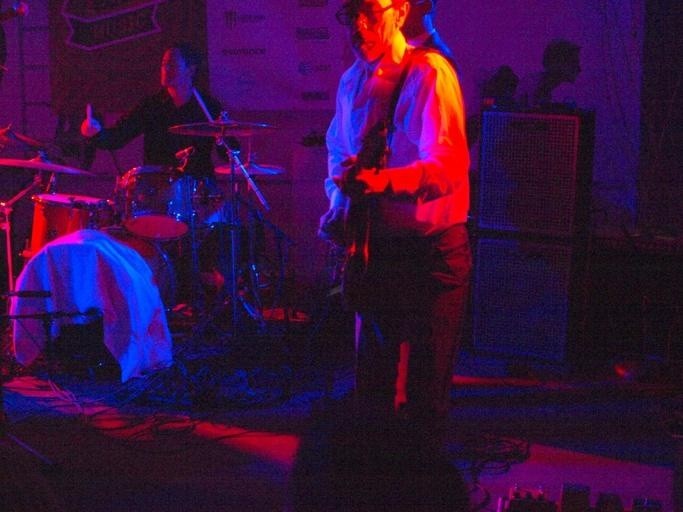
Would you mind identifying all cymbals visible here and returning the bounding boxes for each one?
[169,122,277,138]
[215,164,284,176]
[0,128,44,150]
[0,158,92,176]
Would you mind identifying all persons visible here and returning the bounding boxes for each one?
[318,1,473,416]
[80,36,226,178]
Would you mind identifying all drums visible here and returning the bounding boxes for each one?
[116,164,188,240]
[29,193,114,262]
[102,230,177,324]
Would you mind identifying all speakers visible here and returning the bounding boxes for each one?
[473,103,594,370]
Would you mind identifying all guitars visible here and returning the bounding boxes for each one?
[337,126,390,311]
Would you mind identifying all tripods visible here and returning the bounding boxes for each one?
[185,160,290,343]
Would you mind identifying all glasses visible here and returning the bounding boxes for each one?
[336,0,393,26]
